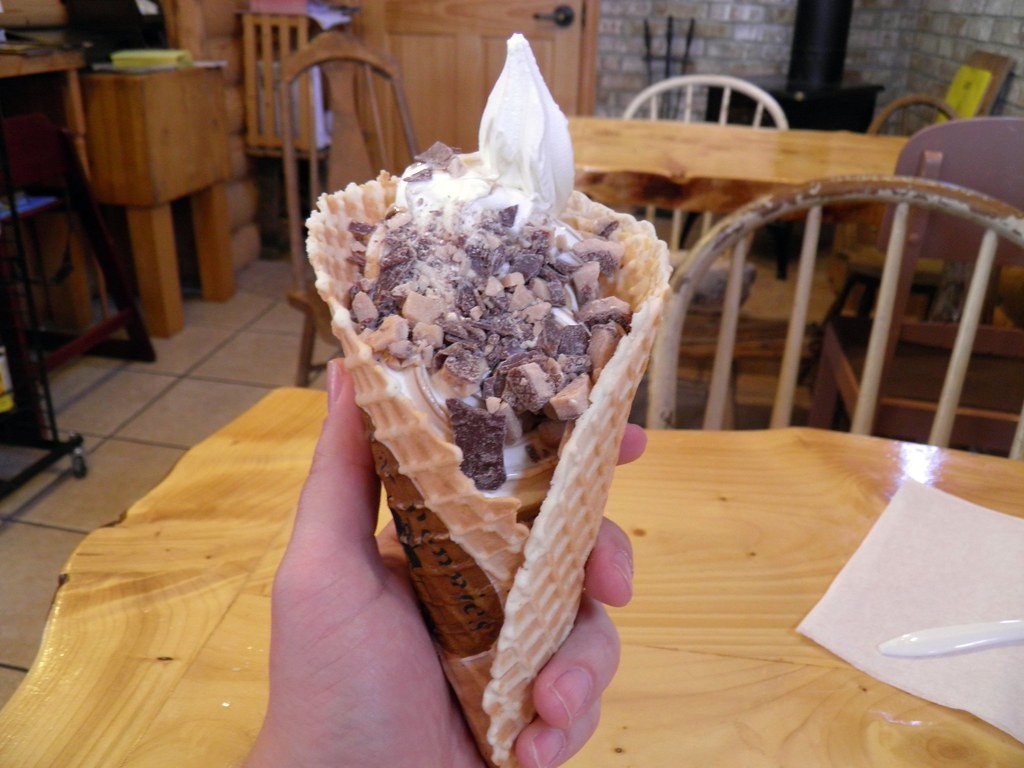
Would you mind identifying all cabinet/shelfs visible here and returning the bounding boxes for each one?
[237,11,347,161]
[0,114,88,501]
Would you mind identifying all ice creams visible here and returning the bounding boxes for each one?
[305,34,676,768]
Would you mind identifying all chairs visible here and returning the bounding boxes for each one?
[0,389,1024,767]
[278,30,422,387]
[620,73,1024,457]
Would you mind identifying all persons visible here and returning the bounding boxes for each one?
[243,358,648,768]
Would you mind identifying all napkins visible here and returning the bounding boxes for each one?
[792,475,1024,746]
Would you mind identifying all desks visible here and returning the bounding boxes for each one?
[0,43,99,324]
[82,67,236,337]
[566,115,935,427]
